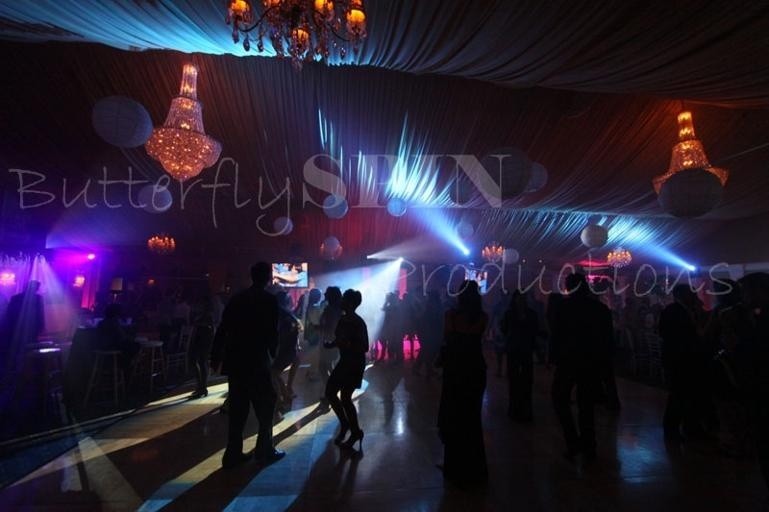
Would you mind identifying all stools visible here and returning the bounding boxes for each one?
[22,321,193,423]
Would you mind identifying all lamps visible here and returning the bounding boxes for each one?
[147,231,176,256]
[145,49,228,182]
[224,1,370,64]
[607,245,632,270]
[650,102,729,223]
[480,239,506,264]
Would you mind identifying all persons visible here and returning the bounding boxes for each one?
[0,260,768,483]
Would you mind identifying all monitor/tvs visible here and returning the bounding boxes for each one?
[463,267,489,296]
[269,261,309,289]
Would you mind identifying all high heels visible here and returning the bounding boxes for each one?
[340,429,363,449]
[188,387,208,399]
[334,421,350,445]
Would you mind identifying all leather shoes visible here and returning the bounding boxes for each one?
[274,452,285,461]
